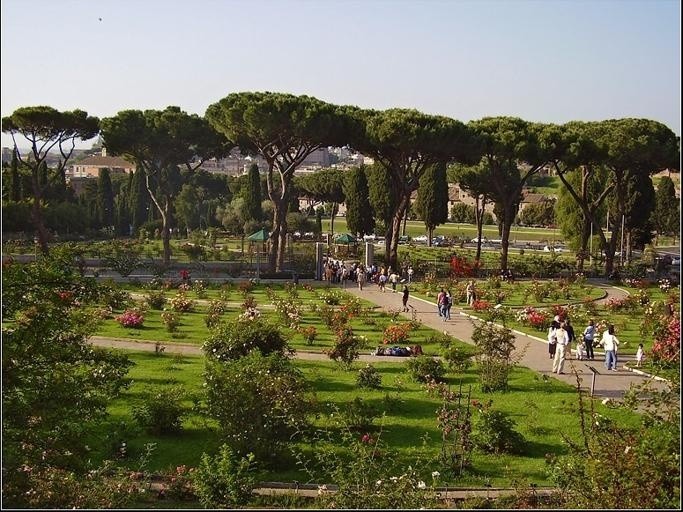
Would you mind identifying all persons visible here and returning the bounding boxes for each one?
[435,288,444,316]
[601,325,617,368]
[546,313,596,376]
[594,328,619,373]
[466,280,476,305]
[445,290,451,319]
[633,343,647,368]
[438,292,447,323]
[468,289,475,307]
[322,243,415,293]
[399,284,409,313]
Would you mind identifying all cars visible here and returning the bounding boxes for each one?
[490,236,502,244]
[470,236,487,243]
[398,234,455,247]
